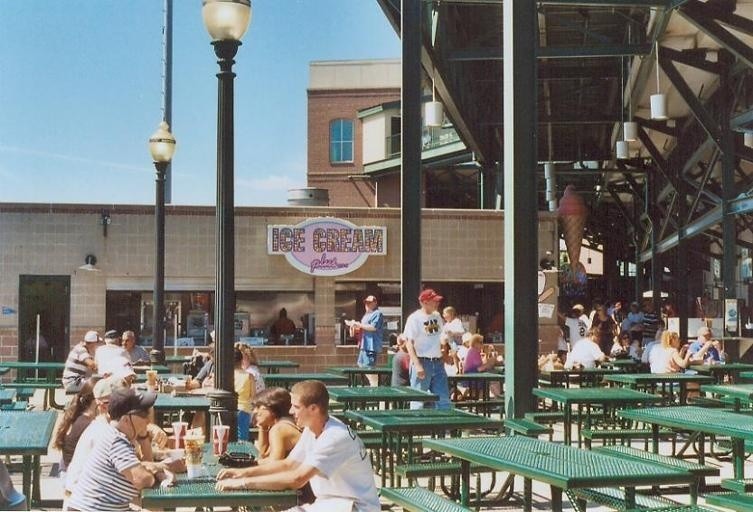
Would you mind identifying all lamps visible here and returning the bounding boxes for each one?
[650,42,669,120]
[617,56,629,159]
[625,25,638,143]
[78,255,102,275]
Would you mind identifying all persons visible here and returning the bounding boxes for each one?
[187,329,266,442]
[270,307,296,335]
[214,380,381,512]
[403,289,452,435]
[344,295,384,387]
[249,387,316,505]
[558,298,730,398]
[52,328,189,512]
[540,251,555,270]
[391,307,504,397]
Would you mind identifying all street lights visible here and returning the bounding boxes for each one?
[147,122,176,365]
[201,0,252,442]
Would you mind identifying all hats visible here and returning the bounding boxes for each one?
[462,332,472,344]
[104,330,122,339]
[84,331,103,343]
[419,289,443,303]
[113,359,137,378]
[363,295,377,303]
[108,387,157,417]
[93,379,129,404]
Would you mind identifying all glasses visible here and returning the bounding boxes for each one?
[125,410,148,418]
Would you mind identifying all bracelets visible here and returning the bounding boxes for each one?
[259,429,268,432]
[241,478,247,491]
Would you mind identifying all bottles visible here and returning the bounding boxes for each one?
[720,351,725,366]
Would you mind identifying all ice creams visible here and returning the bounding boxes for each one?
[559,185,590,280]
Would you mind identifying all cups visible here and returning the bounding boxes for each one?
[187,466,205,479]
[184,375,192,392]
[213,425,230,456]
[183,436,205,465]
[172,422,189,449]
[146,371,157,390]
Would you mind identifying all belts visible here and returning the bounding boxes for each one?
[418,357,439,360]
